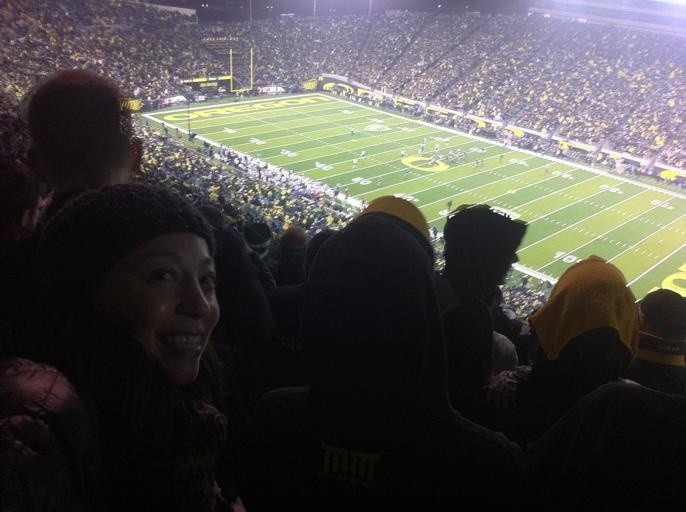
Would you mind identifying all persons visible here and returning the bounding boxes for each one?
[1,195,686,511]
[142,1,686,109]
[138,120,217,197]
[217,143,350,195]
[618,110,686,195]
[400,110,618,172]
[352,158,359,169]
[0,2,140,196]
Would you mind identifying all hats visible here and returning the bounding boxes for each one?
[36,181,221,304]
